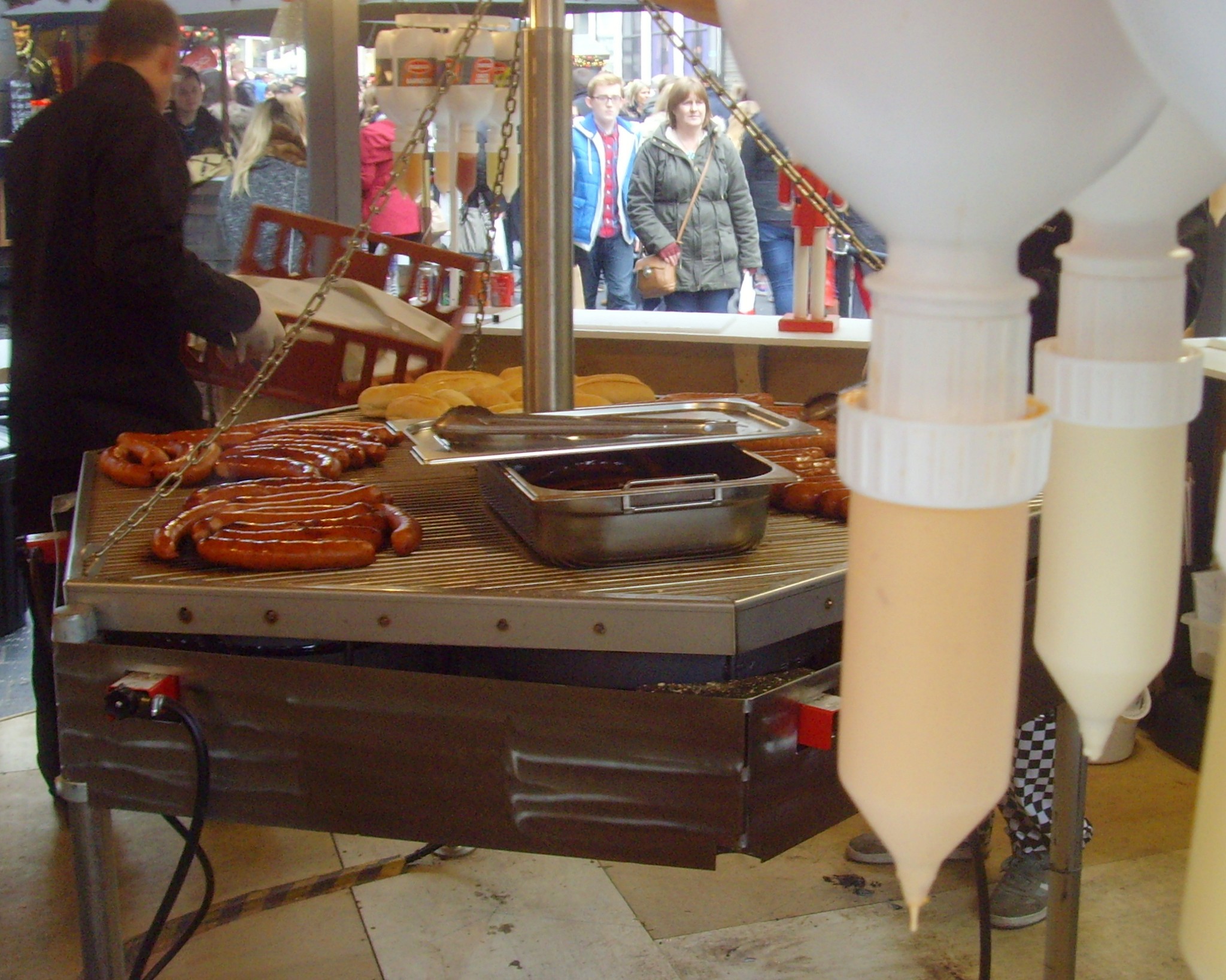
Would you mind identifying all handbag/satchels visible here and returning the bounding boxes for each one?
[633,255,676,299]
[737,270,756,315]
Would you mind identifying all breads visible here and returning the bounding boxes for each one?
[357,365,658,422]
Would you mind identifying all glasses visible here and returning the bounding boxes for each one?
[591,94,623,106]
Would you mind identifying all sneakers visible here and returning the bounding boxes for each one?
[847,829,989,865]
[991,847,1053,928]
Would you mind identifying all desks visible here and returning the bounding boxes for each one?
[488,304,876,419]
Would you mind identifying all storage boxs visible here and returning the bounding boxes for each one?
[201,206,478,415]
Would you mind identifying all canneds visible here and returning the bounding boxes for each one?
[415,261,515,308]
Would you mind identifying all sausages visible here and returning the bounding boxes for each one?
[657,393,851,519]
[150,476,423,571]
[99,417,407,487]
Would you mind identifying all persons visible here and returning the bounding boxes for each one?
[33,28,74,94]
[228,60,256,108]
[196,68,251,148]
[249,70,376,110]
[359,87,424,254]
[9,23,57,101]
[157,63,238,164]
[425,65,888,320]
[214,94,311,279]
[2,0,286,793]
